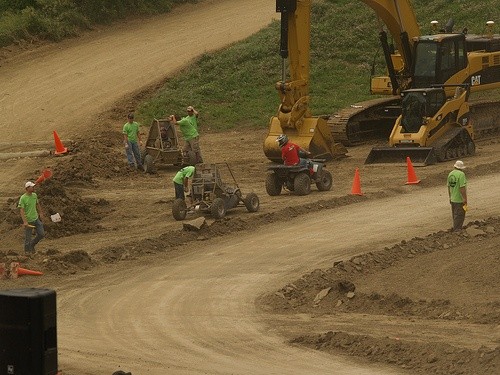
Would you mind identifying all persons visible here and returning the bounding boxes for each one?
[276,134,314,175]
[123,114,147,178]
[185,106,200,156]
[168,114,204,164]
[172,166,195,200]
[447,161,468,230]
[18,181,45,257]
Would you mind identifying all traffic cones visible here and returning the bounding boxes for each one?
[34,168,53,185]
[350,168,364,196]
[9,262,44,278]
[404,155,422,185]
[51,130,68,154]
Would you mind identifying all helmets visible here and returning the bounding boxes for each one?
[276,134,289,148]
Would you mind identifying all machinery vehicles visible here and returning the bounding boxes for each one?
[261,0,500,165]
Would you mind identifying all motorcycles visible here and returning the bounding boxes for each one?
[263,151,333,197]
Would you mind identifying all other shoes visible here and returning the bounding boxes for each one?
[140,166,146,171]
[130,167,136,172]
[25,251,30,255]
[31,249,37,255]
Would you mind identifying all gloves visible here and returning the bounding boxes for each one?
[124,143,129,149]
[140,140,143,146]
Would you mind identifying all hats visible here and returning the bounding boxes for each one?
[187,105,194,111]
[24,181,36,189]
[453,160,467,169]
[128,113,134,117]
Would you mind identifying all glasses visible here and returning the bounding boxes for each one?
[129,118,134,119]
